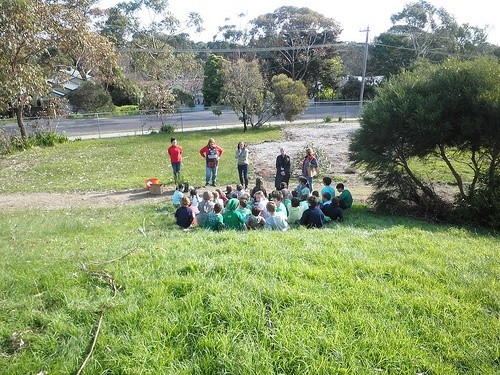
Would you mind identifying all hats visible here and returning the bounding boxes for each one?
[226,198,238,209]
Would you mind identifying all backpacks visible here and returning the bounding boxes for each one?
[206,146,219,168]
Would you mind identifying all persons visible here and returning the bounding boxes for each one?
[235,141,248,191]
[302,148,318,191]
[275,147,290,190]
[167,138,183,182]
[171,176,353,230]
[199,138,223,187]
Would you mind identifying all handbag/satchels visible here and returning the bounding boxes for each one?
[307,163,320,177]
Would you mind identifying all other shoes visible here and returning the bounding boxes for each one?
[244,186,248,191]
[205,183,209,187]
[211,183,216,187]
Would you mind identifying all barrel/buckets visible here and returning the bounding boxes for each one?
[146,178,158,190]
